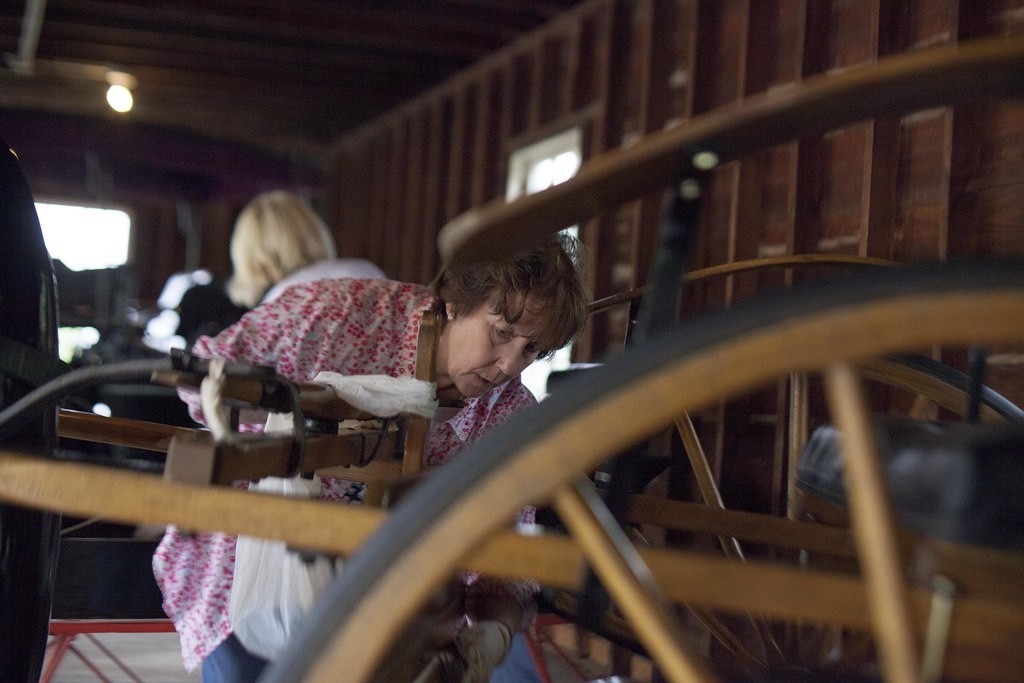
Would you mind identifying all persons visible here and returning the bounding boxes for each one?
[151,232,592,682]
[224,189,385,308]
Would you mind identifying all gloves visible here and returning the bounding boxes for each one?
[459,619,512,683]
